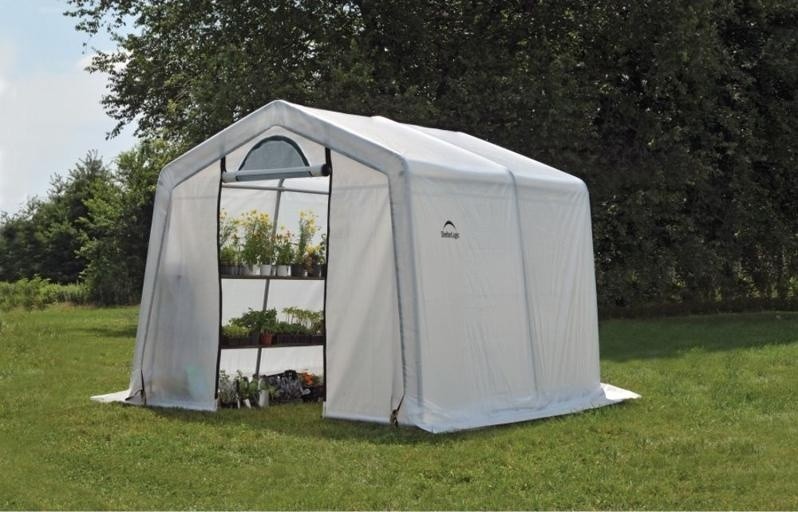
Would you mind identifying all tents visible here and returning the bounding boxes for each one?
[89,99,646,438]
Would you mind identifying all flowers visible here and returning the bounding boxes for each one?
[220,207,327,278]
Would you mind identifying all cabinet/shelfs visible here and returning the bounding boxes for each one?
[219,273,326,408]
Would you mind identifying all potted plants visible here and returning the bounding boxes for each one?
[221,305,325,348]
